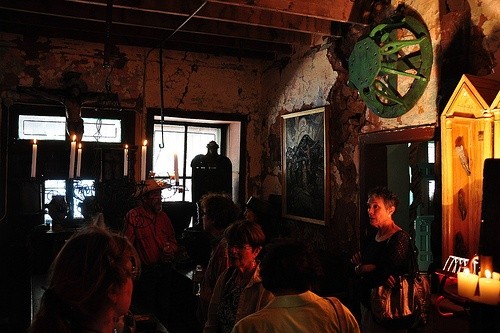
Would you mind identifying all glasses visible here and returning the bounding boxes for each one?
[124,267,136,284]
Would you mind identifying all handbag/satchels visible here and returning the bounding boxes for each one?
[370,230,430,321]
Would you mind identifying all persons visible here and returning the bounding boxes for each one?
[28,228,140,333]
[244,196,269,228]
[122,179,176,267]
[29,84,118,141]
[351,186,418,320]
[195,194,240,301]
[233,238,361,333]
[202,221,274,333]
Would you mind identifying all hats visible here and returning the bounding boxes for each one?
[243,196,264,214]
[132,177,170,196]
